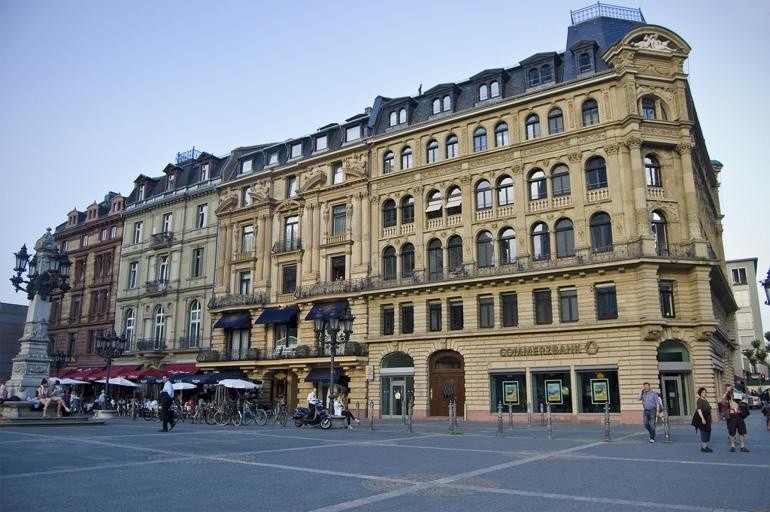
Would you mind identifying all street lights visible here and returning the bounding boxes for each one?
[314,309,356,414]
[96,332,127,396]
[4,228,70,401]
[49,351,69,377]
[761,266,770,306]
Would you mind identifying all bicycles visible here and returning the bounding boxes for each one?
[87,394,292,430]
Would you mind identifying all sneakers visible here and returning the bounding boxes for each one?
[159,426,169,432]
[701,446,713,453]
[649,438,656,444]
[170,421,176,431]
[354,419,361,424]
[740,447,750,453]
[730,447,737,453]
[347,424,354,430]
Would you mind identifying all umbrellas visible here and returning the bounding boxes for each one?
[47,376,263,400]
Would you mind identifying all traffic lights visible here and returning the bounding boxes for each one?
[761,373,765,382]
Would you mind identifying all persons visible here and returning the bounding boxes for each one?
[638,382,660,443]
[696,387,713,453]
[655,392,665,427]
[0,379,6,405]
[334,393,360,429]
[158,375,176,432]
[110,397,208,419]
[36,378,52,418]
[304,387,318,427]
[64,385,71,409]
[99,389,108,410]
[745,387,770,430]
[49,380,71,417]
[336,275,344,281]
[720,383,750,452]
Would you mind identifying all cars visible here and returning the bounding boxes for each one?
[732,386,763,411]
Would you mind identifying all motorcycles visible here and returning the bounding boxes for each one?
[293,398,332,430]
